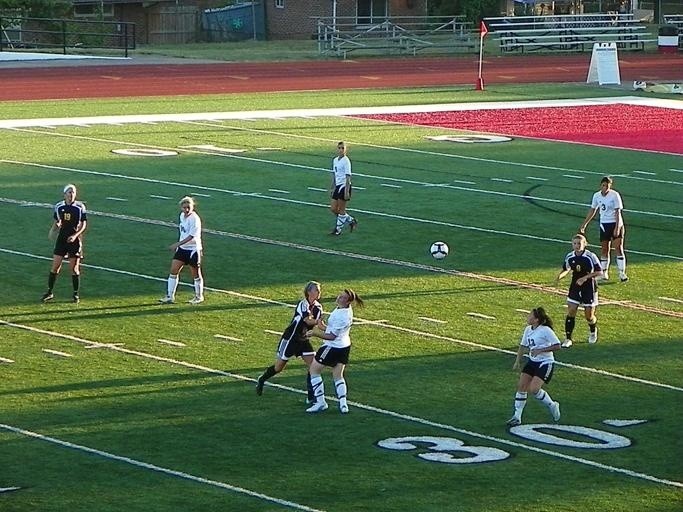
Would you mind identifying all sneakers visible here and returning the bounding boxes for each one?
[552,401,561,421]
[188,295,205,304]
[596,272,609,281]
[158,296,175,303]
[305,397,329,413]
[589,327,598,343]
[504,416,522,425]
[328,230,343,237]
[73,292,80,302]
[256,375,264,396]
[620,273,628,282]
[561,339,573,348]
[350,216,358,232]
[340,405,349,413]
[40,292,54,301]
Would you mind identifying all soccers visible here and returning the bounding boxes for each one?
[430,241,448,259]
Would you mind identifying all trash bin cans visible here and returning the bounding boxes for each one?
[659,26,679,37]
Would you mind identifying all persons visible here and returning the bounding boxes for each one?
[553,233,602,348]
[328,141,357,235]
[254,280,323,404]
[504,307,560,426]
[157,195,204,304]
[304,287,364,413]
[39,183,87,303]
[578,175,628,282]
